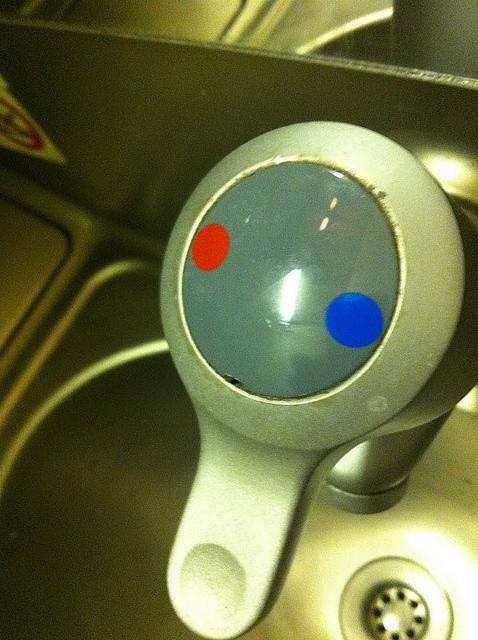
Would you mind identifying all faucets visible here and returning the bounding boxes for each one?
[157,120,478,639]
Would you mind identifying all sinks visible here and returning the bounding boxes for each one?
[0,245,477,638]
[215,0,478,91]
